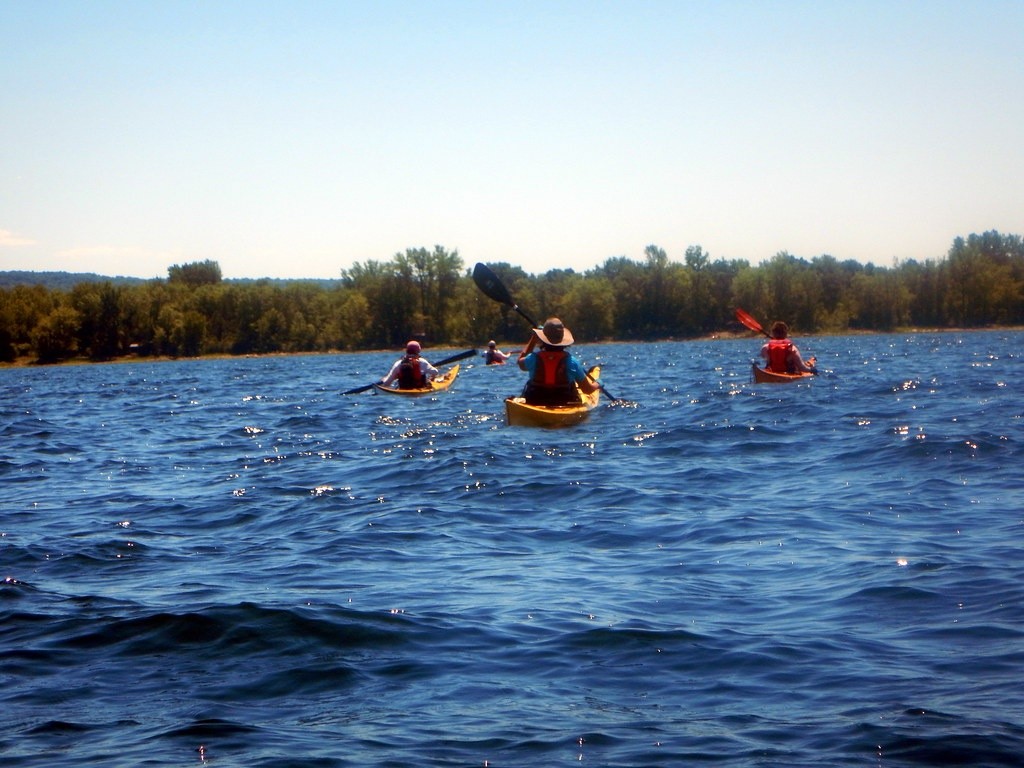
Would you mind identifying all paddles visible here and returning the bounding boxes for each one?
[338,350,478,394]
[502,349,524,356]
[734,306,820,374]
[476,263,618,403]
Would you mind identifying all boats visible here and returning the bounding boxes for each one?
[480,358,506,368]
[749,355,819,384]
[369,363,460,399]
[504,361,604,427]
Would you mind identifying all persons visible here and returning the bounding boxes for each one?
[486,340,511,364]
[518,318,605,407]
[382,341,439,389]
[761,322,818,375]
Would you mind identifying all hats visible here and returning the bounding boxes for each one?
[534,318,574,346]
[772,322,788,335]
[488,341,495,346]
[407,341,420,351]
